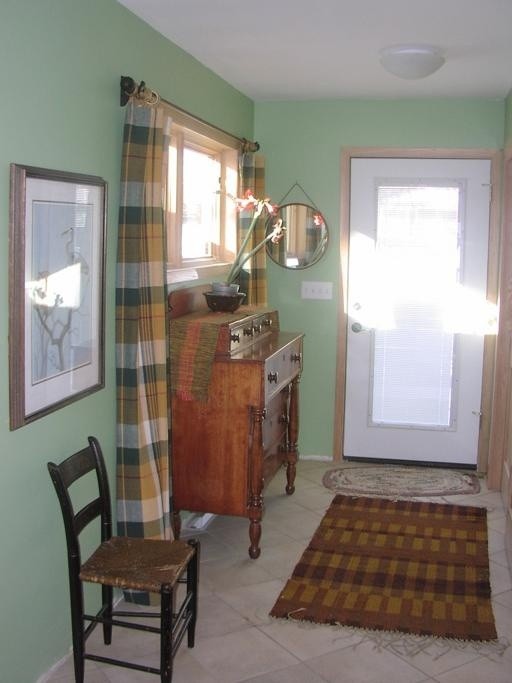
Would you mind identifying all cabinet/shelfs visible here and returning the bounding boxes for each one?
[166,283,307,561]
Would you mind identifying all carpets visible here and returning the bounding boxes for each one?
[322,467,480,498]
[267,492,500,652]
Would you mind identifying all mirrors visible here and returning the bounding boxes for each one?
[264,202,329,271]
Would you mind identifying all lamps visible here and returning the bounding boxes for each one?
[375,46,446,83]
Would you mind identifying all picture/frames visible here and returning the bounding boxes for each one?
[10,161,110,423]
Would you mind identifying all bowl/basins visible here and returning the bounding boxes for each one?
[202,292,246,312]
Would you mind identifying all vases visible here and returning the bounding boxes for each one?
[202,283,247,314]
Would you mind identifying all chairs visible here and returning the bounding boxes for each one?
[47,436,200,682]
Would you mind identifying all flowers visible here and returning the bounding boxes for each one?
[224,190,288,286]
